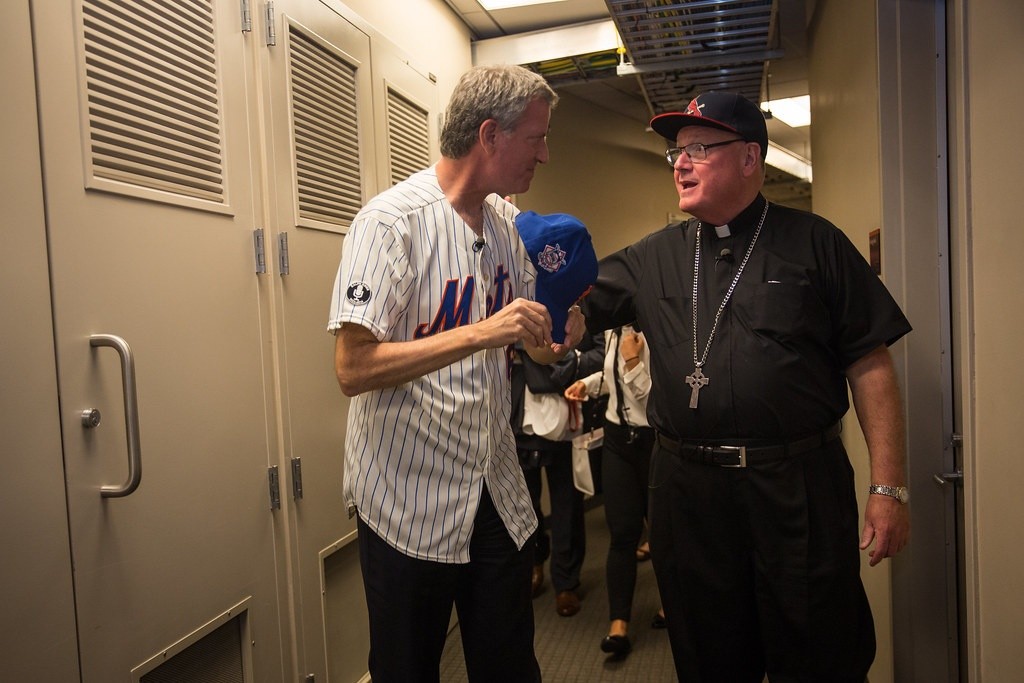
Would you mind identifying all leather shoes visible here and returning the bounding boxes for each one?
[531,549,544,594]
[556,589,578,616]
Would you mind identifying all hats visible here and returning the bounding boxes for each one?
[515,210,598,345]
[650,91,768,160]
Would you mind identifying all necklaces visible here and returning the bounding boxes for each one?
[682,197,770,409]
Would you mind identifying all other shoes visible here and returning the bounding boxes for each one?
[601,634,631,654]
[635,539,651,561]
[652,608,666,629]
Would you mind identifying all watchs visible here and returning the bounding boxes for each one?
[869,484,911,504]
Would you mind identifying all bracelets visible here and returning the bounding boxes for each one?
[625,356,639,362]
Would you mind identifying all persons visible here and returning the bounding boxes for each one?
[326,64,912,683]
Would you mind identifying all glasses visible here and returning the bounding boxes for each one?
[665,138,747,167]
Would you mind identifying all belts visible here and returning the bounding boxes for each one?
[655,422,841,470]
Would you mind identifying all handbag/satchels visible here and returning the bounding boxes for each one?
[571,426,605,497]
